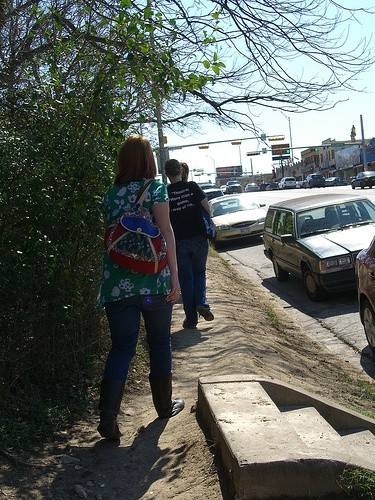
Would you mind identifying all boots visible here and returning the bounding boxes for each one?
[97,378,125,439]
[149,371,184,420]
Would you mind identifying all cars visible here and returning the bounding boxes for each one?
[351,171,375,189]
[325,177,347,187]
[198,181,270,241]
[261,194,375,352]
[266,177,303,191]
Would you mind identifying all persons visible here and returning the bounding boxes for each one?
[165,158,214,328]
[97,134,185,439]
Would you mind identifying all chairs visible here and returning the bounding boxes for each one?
[340,207,359,224]
[325,206,342,224]
[299,215,314,232]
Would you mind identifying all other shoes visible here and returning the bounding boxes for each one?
[183,321,196,329]
[196,305,214,321]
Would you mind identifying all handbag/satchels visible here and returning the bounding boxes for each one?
[104,179,168,274]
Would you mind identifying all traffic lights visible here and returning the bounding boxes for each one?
[310,148,318,151]
[282,149,290,154]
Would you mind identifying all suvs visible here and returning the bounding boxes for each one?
[303,173,325,189]
